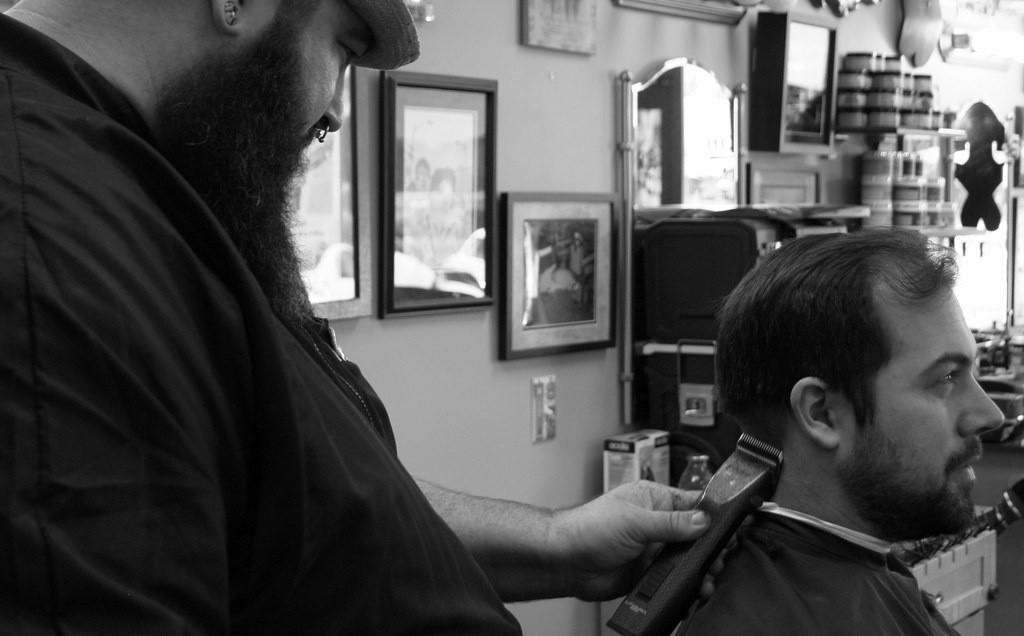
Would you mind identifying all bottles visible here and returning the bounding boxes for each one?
[677,455,714,493]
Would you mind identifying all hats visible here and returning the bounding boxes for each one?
[340,0,419,70]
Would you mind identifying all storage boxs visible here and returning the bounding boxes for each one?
[641,218,849,343]
[644,348,745,486]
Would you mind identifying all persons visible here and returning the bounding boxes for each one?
[671,226,1007,636]
[1,0,764,636]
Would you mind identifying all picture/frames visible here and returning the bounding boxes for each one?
[522,0,599,54]
[284,61,373,325]
[381,69,499,321]
[500,190,618,359]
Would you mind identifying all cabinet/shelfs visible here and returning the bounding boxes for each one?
[836,124,989,242]
